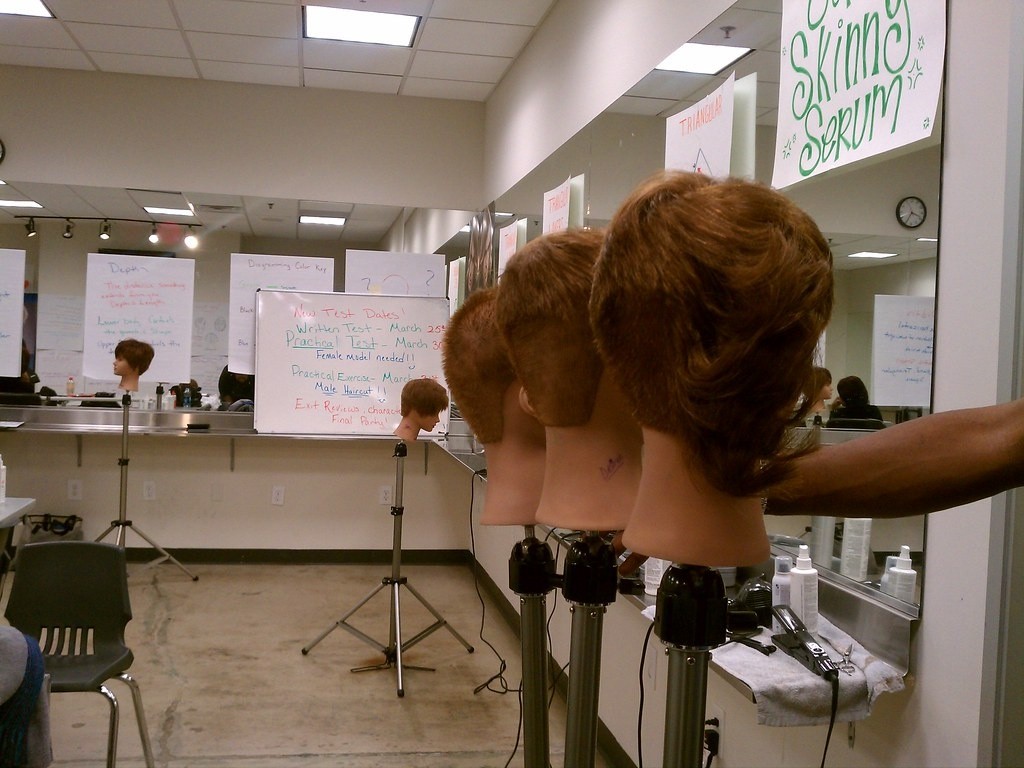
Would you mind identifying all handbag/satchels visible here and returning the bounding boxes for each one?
[2,514,82,572]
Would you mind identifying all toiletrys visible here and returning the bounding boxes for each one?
[879,542,917,606]
[791,542,819,635]
[773,554,791,632]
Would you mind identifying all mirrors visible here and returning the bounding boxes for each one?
[0,0,949,617]
[491,210,937,433]
[430,222,473,421]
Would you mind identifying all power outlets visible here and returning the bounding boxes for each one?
[143,481,156,501]
[67,479,82,500]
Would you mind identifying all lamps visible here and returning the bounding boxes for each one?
[148,222,159,243]
[99,219,111,240]
[62,219,76,238]
[24,218,36,237]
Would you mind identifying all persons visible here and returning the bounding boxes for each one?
[828,376,882,422]
[393,378,449,441]
[218,365,255,404]
[759,397,1024,519]
[441,287,546,526]
[587,168,834,567]
[112,339,154,391]
[802,367,833,410]
[493,228,643,531]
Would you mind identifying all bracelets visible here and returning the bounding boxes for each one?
[759,457,768,513]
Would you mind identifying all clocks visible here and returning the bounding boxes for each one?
[896,196,926,229]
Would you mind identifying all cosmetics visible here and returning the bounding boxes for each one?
[879,545,917,604]
[772,545,818,633]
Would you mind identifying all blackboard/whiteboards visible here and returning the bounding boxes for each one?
[253,288,450,439]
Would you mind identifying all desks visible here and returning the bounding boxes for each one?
[0,498,37,556]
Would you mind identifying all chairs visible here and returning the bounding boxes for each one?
[4,541,157,768]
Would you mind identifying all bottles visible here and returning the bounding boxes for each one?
[773,554,793,633]
[791,544,818,638]
[811,515,915,607]
[66,376,75,394]
[0,454,6,502]
[183,388,191,407]
